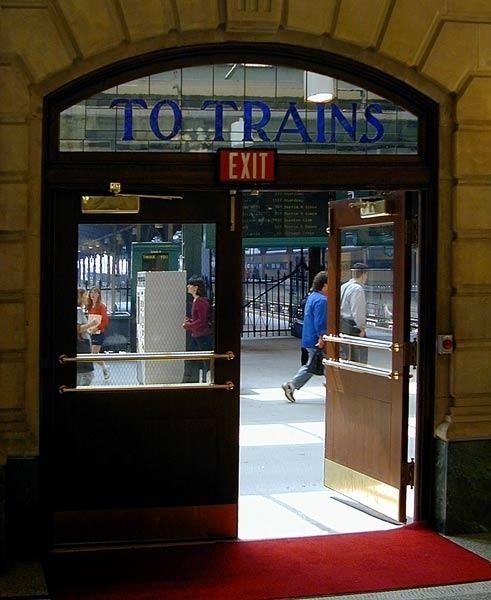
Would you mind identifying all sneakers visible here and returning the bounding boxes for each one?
[282,382,295,402]
[102,365,110,380]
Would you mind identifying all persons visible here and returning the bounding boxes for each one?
[340,262,370,368]
[183,276,215,383]
[283,270,347,402]
[77,288,99,386]
[85,288,109,379]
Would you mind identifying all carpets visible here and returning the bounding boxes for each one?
[35,518,491,600]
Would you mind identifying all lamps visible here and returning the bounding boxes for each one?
[300,66,338,105]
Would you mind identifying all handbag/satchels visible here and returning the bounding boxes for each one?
[300,345,324,375]
